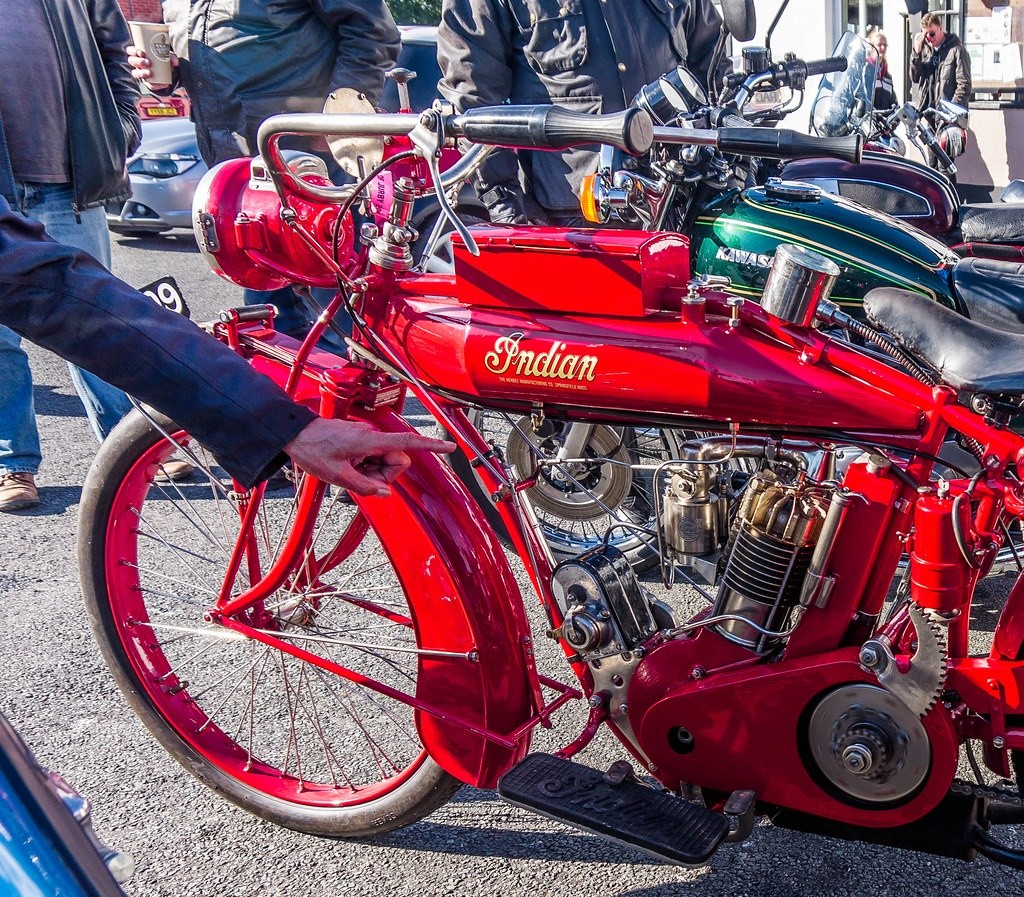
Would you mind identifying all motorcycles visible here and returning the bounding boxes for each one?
[0,0,1024,897]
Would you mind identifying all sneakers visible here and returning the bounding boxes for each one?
[0,472,40,511]
[154,456,195,481]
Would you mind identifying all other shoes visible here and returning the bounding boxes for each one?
[265,464,294,491]
[330,484,351,502]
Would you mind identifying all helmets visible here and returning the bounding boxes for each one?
[879,133,906,157]
[938,124,967,160]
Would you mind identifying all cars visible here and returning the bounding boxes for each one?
[105,116,210,238]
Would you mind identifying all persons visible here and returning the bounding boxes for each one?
[0,0,197,512]
[2,195,457,498]
[908,12,972,192]
[858,31,901,145]
[162,0,404,499]
[437,0,725,524]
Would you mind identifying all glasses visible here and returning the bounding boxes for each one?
[874,44,888,48]
[924,25,938,38]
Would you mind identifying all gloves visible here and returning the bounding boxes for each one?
[727,164,749,190]
[478,184,551,226]
